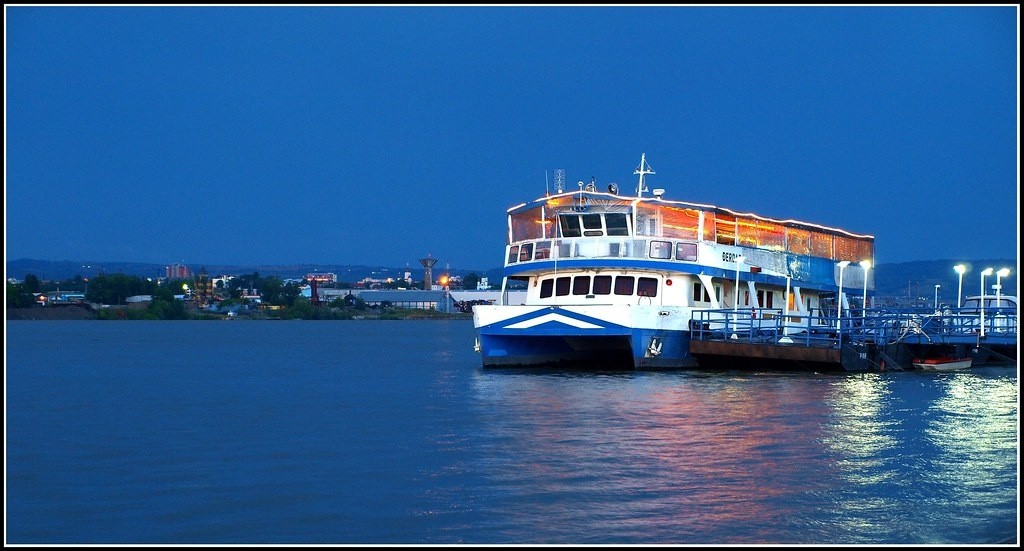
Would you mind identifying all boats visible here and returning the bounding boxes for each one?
[912,357,973,371]
[956,296,1017,334]
[472,152,876,369]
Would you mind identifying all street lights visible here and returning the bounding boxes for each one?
[953,265,965,332]
[997,268,1009,332]
[934,284,940,313]
[980,268,993,336]
[834,261,850,345]
[730,256,746,339]
[860,260,871,335]
